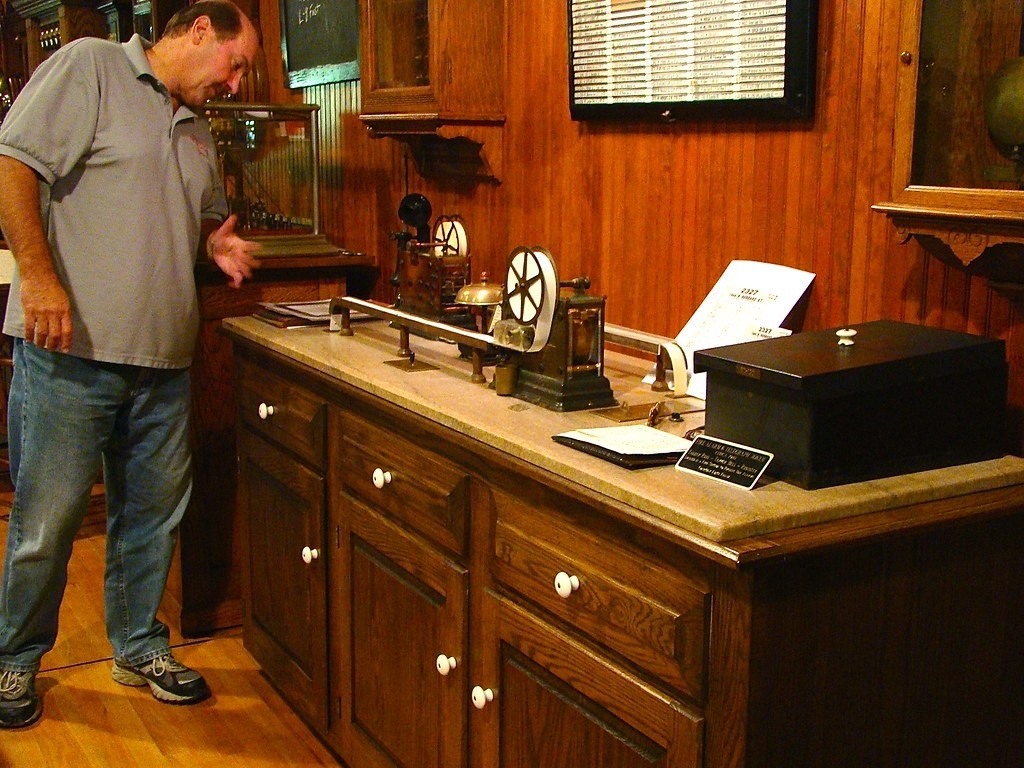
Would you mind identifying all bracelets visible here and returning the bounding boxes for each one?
[207,238,214,260]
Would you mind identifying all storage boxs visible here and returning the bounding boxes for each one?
[694,318,1010,492]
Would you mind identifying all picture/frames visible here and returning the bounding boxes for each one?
[279,0,362,89]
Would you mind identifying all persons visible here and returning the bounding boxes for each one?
[0,0,261,728]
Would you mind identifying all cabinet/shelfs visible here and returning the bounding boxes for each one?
[158,253,374,639]
[215,309,1024,768]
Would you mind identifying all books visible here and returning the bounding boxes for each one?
[252,296,395,327]
[552,423,694,471]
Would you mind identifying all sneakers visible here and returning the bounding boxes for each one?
[0,669,42,726]
[111,653,204,703]
